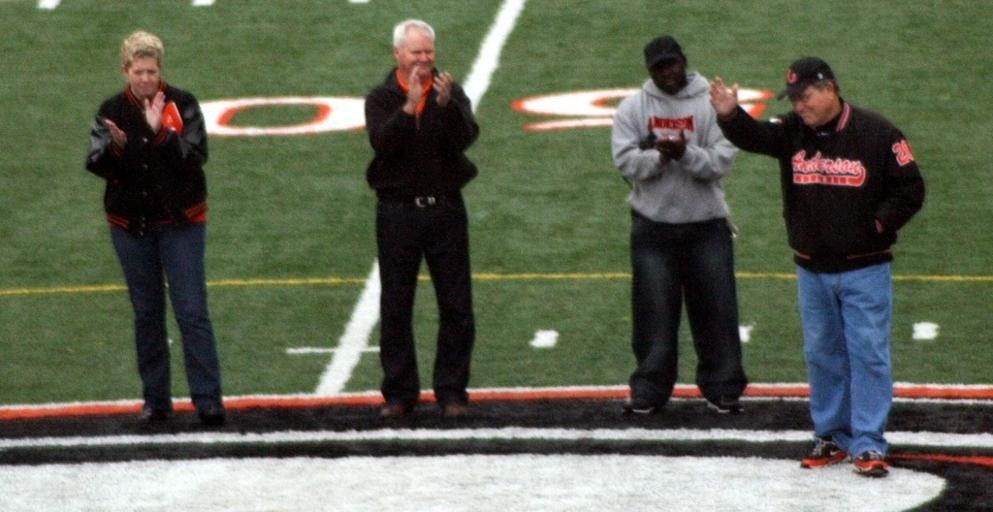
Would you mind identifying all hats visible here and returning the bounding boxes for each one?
[776,56,837,101]
[644,36,683,71]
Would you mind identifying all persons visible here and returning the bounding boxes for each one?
[85,27,226,426]
[704,54,927,478]
[361,17,484,422]
[609,32,751,418]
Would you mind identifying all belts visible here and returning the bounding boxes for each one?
[405,195,444,208]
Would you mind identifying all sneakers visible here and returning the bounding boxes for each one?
[632,396,654,415]
[707,392,745,414]
[852,451,890,476]
[800,438,853,470]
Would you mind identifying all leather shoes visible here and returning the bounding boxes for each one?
[137,403,156,422]
[439,398,469,419]
[381,401,405,418]
[197,402,227,421]
